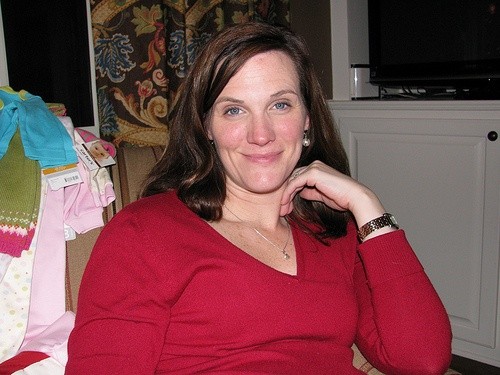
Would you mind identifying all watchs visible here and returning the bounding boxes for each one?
[357,213,401,242]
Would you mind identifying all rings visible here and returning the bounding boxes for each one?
[294,172,301,180]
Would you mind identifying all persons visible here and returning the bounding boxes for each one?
[65,22,453,374]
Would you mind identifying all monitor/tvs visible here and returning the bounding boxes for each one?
[366,0,500,92]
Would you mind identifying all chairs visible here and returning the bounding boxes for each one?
[60,143,165,314]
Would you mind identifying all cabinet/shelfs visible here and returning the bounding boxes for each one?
[338,115,499,349]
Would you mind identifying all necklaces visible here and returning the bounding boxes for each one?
[225,203,293,262]
